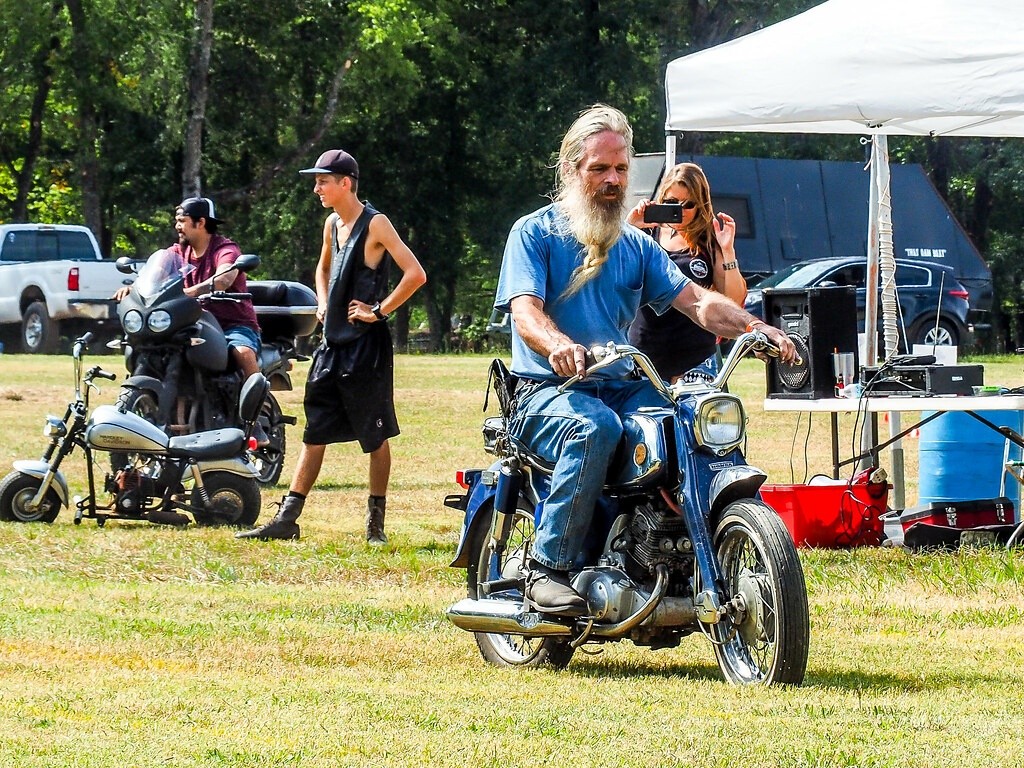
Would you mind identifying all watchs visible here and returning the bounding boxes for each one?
[369,301,389,320]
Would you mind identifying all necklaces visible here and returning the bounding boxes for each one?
[666,222,685,240]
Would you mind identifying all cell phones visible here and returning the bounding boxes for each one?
[643,204,682,224]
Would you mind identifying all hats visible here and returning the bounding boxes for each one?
[175,197,225,225]
[299,150,359,180]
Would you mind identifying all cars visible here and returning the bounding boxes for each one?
[718,253,971,357]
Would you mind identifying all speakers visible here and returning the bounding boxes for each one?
[759,284,860,401]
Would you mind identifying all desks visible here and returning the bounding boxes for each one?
[763,392,1024,515]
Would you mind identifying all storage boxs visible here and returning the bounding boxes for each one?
[900,498,1015,538]
[759,483,893,550]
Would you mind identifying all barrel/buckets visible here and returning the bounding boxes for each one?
[917,409,1024,527]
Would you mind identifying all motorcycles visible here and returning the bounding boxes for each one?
[444,330,811,692]
[105,252,321,488]
[0,330,273,530]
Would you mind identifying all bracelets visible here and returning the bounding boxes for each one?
[722,259,738,271]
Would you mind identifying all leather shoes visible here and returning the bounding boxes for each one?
[523,558,587,617]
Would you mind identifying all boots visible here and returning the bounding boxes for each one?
[236,496,305,539]
[364,496,388,546]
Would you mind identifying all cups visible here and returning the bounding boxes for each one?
[834,352,855,386]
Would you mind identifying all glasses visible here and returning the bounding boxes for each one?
[663,197,698,210]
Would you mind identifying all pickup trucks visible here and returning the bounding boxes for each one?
[0,222,152,355]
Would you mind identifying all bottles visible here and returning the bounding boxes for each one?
[836,373,845,398]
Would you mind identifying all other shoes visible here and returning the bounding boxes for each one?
[253,422,271,446]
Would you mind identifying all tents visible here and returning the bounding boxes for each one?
[665,0,1024,512]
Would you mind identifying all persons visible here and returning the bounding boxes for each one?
[486,102,801,615]
[625,162,748,393]
[113,197,270,448]
[235,149,426,548]
